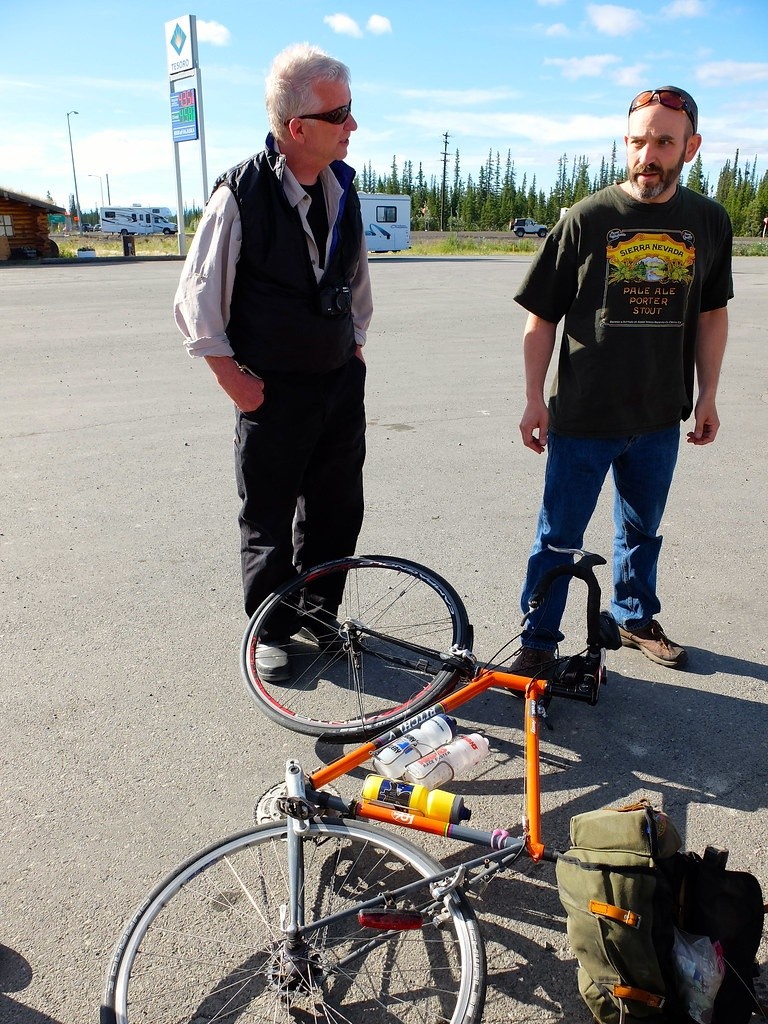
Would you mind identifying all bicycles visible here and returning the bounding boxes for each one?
[96,543,625,1024]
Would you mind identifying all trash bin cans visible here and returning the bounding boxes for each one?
[123,235,135,256]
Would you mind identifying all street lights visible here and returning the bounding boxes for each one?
[66,110,85,231]
[88,174,104,206]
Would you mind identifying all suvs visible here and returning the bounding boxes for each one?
[509,218,548,238]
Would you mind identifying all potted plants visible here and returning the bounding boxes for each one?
[77,247,96,257]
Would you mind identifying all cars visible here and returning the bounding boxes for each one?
[93,224,102,232]
[83,223,94,232]
[63,225,89,232]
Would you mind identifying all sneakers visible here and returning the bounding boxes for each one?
[615,622,689,668]
[297,597,356,653]
[254,628,290,683]
[508,645,557,692]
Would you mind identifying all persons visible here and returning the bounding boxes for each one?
[174,46,373,682]
[510,85,735,695]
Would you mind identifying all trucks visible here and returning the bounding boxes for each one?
[99,206,178,236]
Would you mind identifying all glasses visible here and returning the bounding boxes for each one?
[627,90,698,133]
[283,99,352,125]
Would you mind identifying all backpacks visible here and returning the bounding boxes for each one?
[557,804,764,1023]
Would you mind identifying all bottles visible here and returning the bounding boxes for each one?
[361,774,471,825]
[372,713,457,779]
[405,733,489,785]
